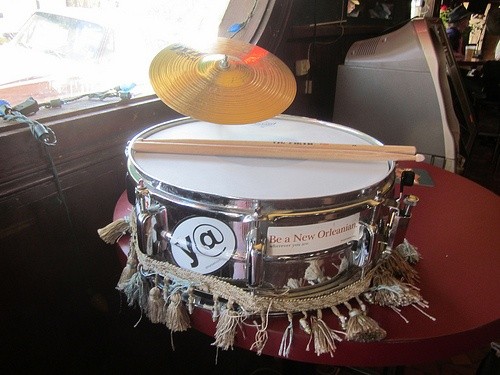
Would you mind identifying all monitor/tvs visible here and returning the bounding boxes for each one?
[335,16,475,173]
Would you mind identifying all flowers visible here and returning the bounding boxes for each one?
[440,4,449,29]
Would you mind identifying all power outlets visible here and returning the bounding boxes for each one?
[296,59,310,76]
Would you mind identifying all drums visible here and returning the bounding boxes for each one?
[124,114,419,315]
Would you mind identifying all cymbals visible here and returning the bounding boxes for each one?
[150,36,296,126]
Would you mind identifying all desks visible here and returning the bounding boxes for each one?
[112,161,500,375]
